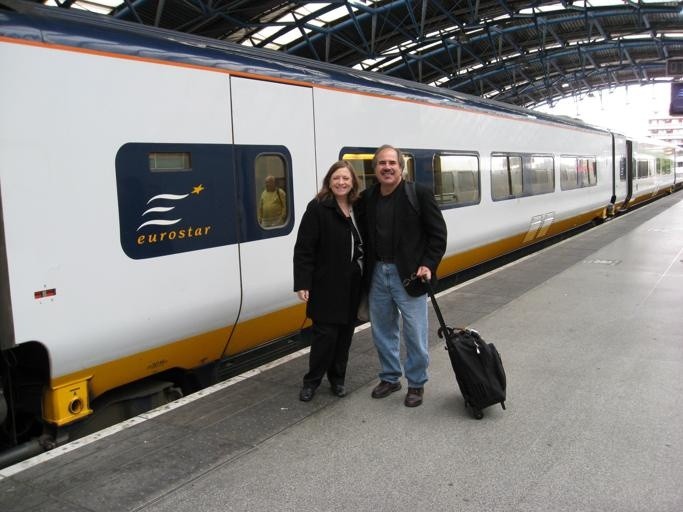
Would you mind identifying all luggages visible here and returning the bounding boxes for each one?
[421,274,506,420]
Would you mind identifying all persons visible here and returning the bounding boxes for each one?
[257,176,286,227]
[291,160,367,401]
[350,144,448,407]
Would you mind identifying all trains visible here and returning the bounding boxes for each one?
[0,0,683,477]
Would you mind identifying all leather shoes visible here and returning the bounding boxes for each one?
[333,385,346,397]
[299,388,313,401]
[405,388,424,407]
[372,381,401,398]
